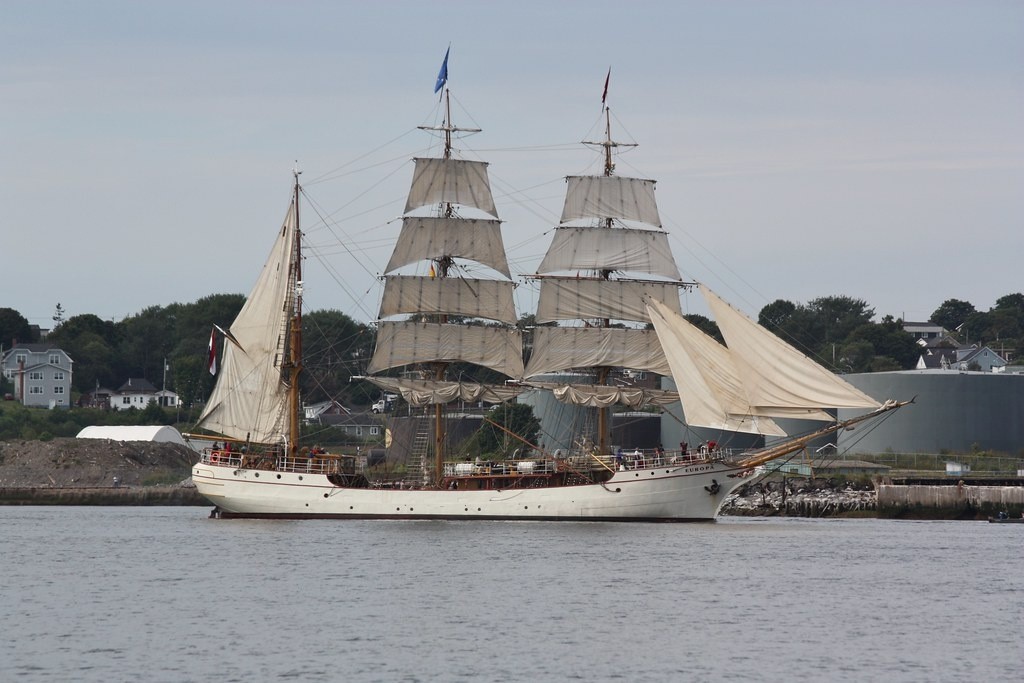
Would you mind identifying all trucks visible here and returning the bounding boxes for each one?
[372,394,398,414]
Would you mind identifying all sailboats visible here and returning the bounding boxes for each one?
[181,42,919,522]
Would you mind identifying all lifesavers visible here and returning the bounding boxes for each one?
[211,453,219,462]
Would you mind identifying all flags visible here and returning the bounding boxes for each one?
[435,45,450,94]
[429,260,435,282]
[602,66,611,102]
[209,327,217,376]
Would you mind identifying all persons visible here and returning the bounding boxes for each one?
[300,445,330,474]
[465,453,471,464]
[212,441,250,466]
[680,440,719,463]
[616,444,665,469]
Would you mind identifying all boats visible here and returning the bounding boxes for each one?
[988,516,1024,523]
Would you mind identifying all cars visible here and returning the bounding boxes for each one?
[4,393,13,401]
